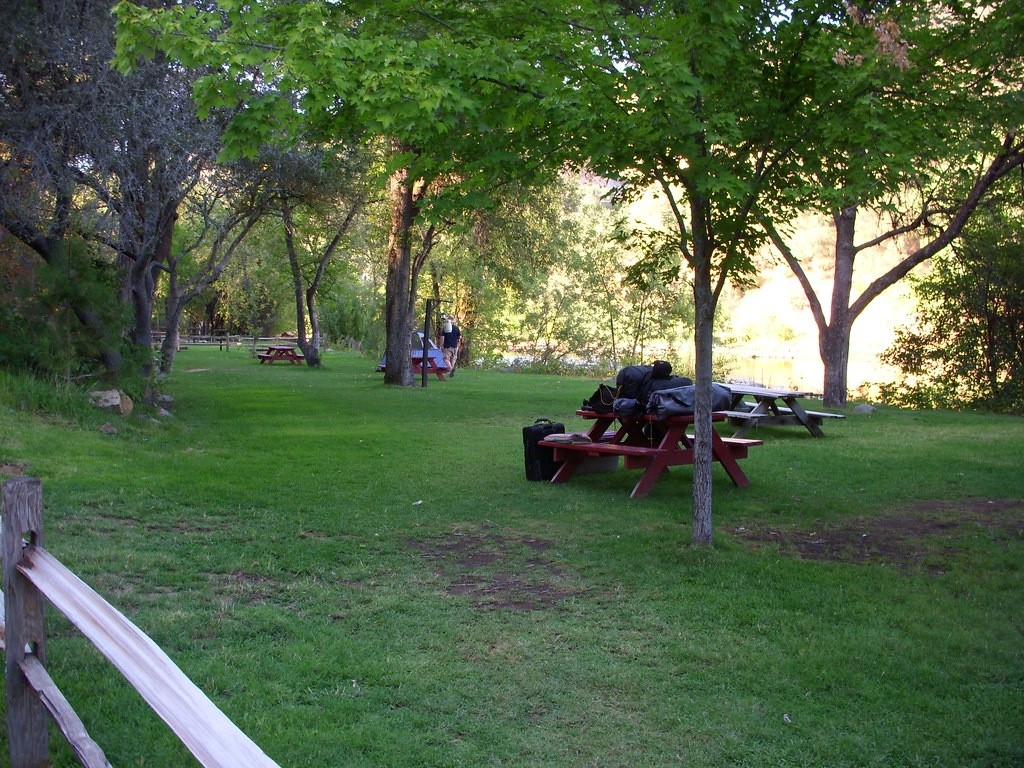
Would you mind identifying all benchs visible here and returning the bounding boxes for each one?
[427,366,450,381]
[714,410,768,438]
[686,434,763,488]
[745,401,846,438]
[538,440,667,500]
[257,354,273,366]
[289,354,305,366]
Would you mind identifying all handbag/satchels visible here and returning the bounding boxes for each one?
[588,357,733,420]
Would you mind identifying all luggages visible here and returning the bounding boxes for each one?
[522,417,565,481]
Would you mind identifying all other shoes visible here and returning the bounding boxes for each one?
[450,368,455,377]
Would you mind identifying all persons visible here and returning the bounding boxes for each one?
[434,314,464,377]
[436,316,464,366]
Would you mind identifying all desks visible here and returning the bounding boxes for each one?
[550,409,728,499]
[720,383,825,441]
[259,345,302,366]
[411,356,445,381]
